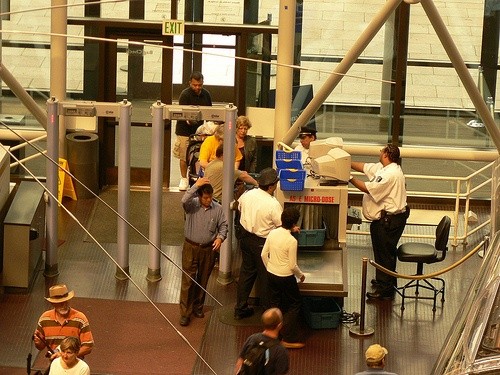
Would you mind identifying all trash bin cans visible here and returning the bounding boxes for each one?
[0,114,25,177]
[65,132,100,198]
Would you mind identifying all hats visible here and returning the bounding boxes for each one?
[43,284,74,303]
[297,126,318,138]
[365,343,388,362]
[258,168,280,186]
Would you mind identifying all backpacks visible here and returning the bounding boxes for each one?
[237,334,279,375]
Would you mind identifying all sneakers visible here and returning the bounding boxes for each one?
[179,177,188,191]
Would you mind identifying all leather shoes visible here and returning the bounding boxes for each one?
[371,279,379,286]
[367,291,395,300]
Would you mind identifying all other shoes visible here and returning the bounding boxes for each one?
[233,306,254,320]
[179,316,190,326]
[280,339,305,348]
[192,309,204,318]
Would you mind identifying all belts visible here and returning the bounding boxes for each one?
[185,237,214,247]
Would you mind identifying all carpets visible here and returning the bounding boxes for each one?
[354,206,456,227]
[83,190,186,246]
[30,297,214,375]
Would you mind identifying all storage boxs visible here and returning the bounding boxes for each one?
[275,150,306,191]
[291,217,327,246]
[303,296,342,329]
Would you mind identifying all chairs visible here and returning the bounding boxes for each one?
[394,216,451,312]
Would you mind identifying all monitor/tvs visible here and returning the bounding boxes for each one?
[308,137,352,184]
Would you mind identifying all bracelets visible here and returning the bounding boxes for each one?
[349,175,353,182]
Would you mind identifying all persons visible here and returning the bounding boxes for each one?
[236,116,258,174]
[199,124,259,205]
[295,127,318,164]
[349,144,410,299]
[179,178,228,325]
[260,208,305,348]
[233,168,301,320]
[34,285,94,360]
[355,344,396,375]
[49,337,90,375]
[173,72,212,191]
[235,308,289,375]
